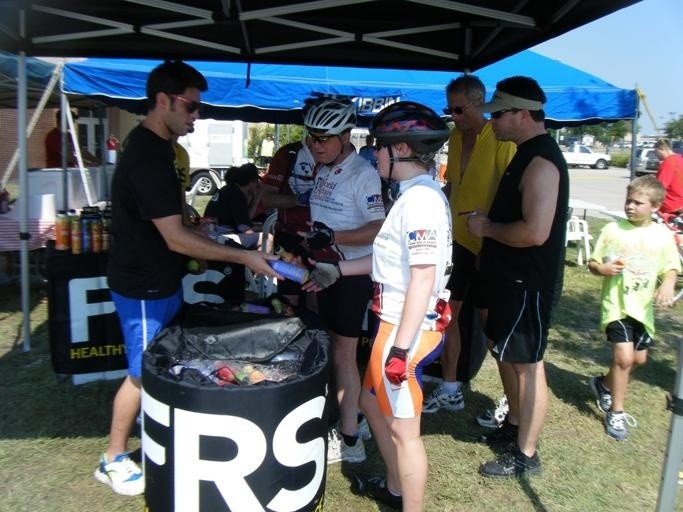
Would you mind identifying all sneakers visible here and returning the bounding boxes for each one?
[477,420,519,447]
[329,413,372,443]
[327,429,366,465]
[477,444,542,481]
[589,374,612,414]
[421,385,466,414]
[93,451,145,497]
[604,409,638,441]
[351,471,402,510]
[477,394,510,429]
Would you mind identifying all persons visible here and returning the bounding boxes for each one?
[94,62,284,495]
[297,98,386,464]
[465,77,569,478]
[653,139,683,229]
[203,162,260,237]
[587,178,681,440]
[254,133,276,168]
[45,108,101,168]
[421,75,517,428]
[301,101,454,512]
[254,97,331,244]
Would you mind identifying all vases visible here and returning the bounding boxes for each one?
[0,200,9,214]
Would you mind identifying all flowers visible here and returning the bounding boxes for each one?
[0,188,9,200]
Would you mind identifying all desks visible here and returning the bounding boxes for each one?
[46,238,246,386]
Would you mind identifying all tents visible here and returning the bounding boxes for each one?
[60,48,639,180]
[0,53,60,91]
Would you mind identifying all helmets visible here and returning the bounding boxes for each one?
[368,100,453,164]
[301,95,357,138]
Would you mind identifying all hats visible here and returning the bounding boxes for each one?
[473,91,545,117]
[239,162,264,185]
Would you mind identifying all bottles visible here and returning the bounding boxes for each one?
[92,219,102,252]
[55,210,70,251]
[82,220,91,251]
[70,215,81,253]
[275,246,304,269]
[267,260,309,284]
[102,218,112,250]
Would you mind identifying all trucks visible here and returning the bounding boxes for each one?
[177,119,248,196]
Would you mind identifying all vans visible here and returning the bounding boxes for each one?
[627,148,661,177]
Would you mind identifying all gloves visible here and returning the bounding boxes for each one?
[309,260,341,288]
[384,344,410,386]
[292,245,311,267]
[295,188,312,206]
[303,222,337,253]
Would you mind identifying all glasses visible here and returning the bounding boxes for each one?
[490,108,517,120]
[442,102,474,115]
[373,140,403,151]
[171,92,203,114]
[307,132,336,144]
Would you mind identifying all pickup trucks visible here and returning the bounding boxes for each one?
[562,145,612,170]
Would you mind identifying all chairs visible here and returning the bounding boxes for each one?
[564,216,593,266]
